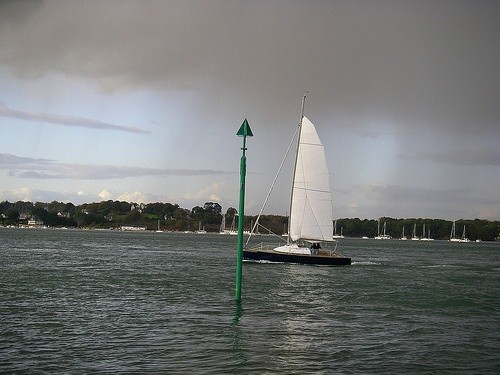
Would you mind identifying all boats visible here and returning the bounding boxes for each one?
[195,230,207,235]
[362,236,368,239]
[475,239,482,242]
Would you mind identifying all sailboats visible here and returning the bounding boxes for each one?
[380,222,392,240]
[374,220,382,240]
[399,226,407,241]
[333,220,345,238]
[249,219,261,236]
[227,215,238,236]
[281,223,288,237]
[421,230,434,241]
[449,217,461,242]
[241,90,352,266]
[411,223,419,240]
[219,213,229,235]
[458,225,470,243]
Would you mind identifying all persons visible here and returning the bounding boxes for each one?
[310,243,322,255]
